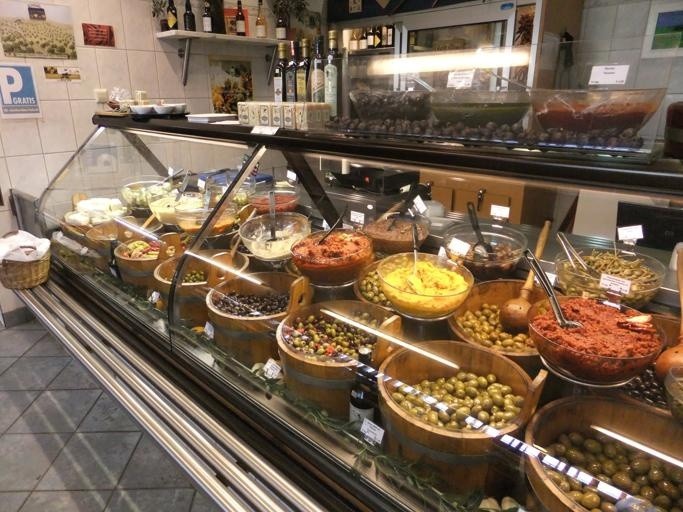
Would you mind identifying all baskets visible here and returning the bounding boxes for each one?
[0,230,50,289]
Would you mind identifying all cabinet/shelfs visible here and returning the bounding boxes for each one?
[330,0,584,132]
[419,171,557,231]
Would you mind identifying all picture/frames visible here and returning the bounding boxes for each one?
[641,0,682,58]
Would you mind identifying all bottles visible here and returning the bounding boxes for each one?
[347,347,378,432]
[182,0,197,32]
[235,0,246,36]
[273,23,395,120]
[202,7,213,32]
[256,0,267,38]
[166,0,180,31]
[275,6,287,39]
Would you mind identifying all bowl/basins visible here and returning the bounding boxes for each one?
[430,89,532,128]
[554,248,668,310]
[119,169,299,236]
[377,253,472,321]
[529,87,668,136]
[347,89,432,123]
[525,296,668,389]
[239,213,311,262]
[129,103,187,113]
[290,228,373,284]
[442,221,528,278]
[364,211,432,253]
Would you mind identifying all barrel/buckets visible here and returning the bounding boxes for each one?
[85,216,137,260]
[154,248,250,319]
[455,280,565,375]
[113,232,183,286]
[590,318,683,415]
[522,394,683,512]
[62,192,91,239]
[275,298,403,430]
[205,270,313,367]
[378,339,548,502]
